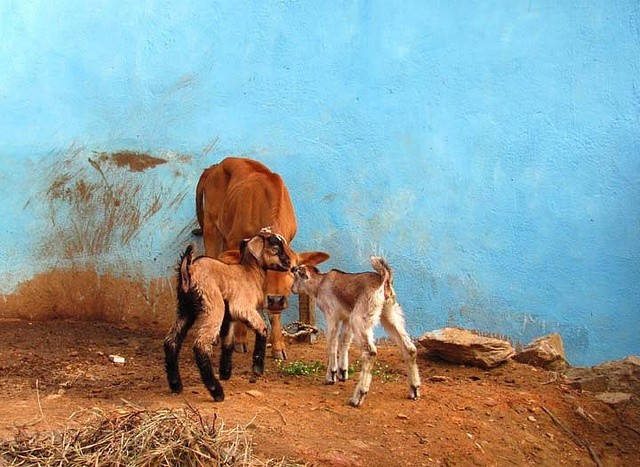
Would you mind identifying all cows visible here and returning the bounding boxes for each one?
[191,156,331,360]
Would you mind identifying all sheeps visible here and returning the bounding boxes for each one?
[163,228,295,403]
[290,253,424,408]
